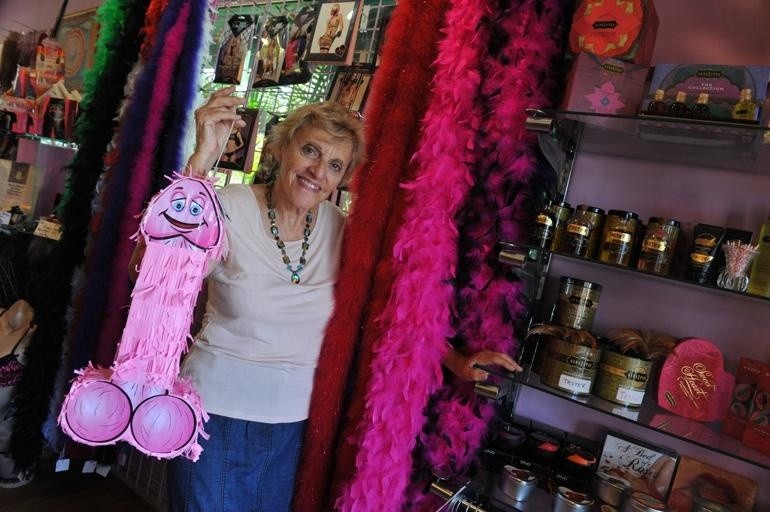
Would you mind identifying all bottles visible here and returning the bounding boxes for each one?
[649,89,712,120]
[732,88,758,122]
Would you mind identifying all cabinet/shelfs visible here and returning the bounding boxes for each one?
[429,108,770,512]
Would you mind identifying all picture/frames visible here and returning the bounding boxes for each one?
[325,63,373,119]
[303,0,365,66]
[56,7,100,92]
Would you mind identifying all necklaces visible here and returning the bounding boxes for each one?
[263,181,314,284]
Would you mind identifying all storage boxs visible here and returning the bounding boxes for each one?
[1,132,78,232]
[560,0,770,124]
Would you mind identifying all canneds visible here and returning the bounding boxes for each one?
[552,471,666,512]
[497,464,538,502]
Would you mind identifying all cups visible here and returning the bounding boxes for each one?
[715,243,760,293]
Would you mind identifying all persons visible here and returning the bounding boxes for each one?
[222,125,245,163]
[223,29,302,78]
[166,86,524,512]
[319,4,343,53]
